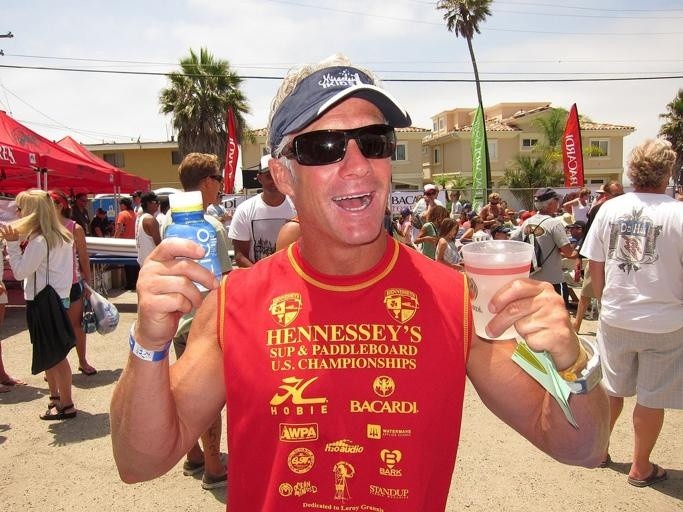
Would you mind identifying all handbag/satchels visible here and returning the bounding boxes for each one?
[81,311,98,333]
[27,285,77,374]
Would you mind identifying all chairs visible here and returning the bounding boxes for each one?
[558,257,600,320]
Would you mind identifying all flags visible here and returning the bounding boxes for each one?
[223,105,239,195]
[472,102,488,212]
[562,104,584,188]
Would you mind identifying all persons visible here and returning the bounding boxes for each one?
[108,52,610,512]
[159,152,234,490]
[580,137,682,487]
[50,153,300,375]
[383,179,626,312]
[0,186,79,421]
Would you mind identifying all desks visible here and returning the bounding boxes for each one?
[77,253,139,301]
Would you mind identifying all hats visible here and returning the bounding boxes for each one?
[259,153,272,175]
[130,191,143,197]
[401,186,605,233]
[268,67,412,151]
[95,208,107,215]
[218,191,224,196]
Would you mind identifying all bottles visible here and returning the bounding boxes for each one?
[162,191,223,290]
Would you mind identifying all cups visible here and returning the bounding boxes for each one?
[461,239,536,342]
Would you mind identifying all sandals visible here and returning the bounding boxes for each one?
[39,397,76,419]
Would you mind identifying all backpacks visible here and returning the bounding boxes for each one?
[523,216,556,275]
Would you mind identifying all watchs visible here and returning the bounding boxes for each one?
[564,339,604,394]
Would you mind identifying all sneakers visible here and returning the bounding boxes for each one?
[183,452,227,487]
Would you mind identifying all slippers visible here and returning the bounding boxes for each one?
[78,366,96,375]
[1,383,11,393]
[2,376,28,386]
[628,463,668,487]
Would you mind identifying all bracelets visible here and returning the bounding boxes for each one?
[556,341,588,380]
[127,319,174,362]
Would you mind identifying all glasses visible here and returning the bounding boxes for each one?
[277,123,397,166]
[210,175,223,182]
[151,200,160,204]
